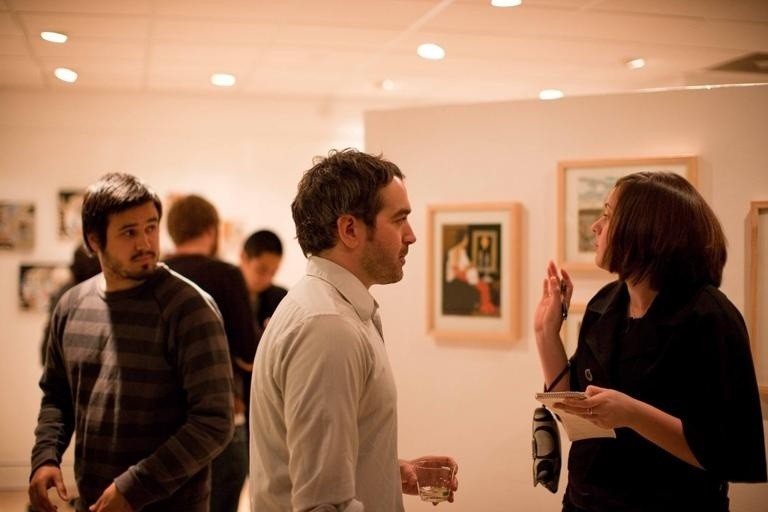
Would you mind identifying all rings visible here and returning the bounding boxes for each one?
[585,408,593,416]
[31,492,37,499]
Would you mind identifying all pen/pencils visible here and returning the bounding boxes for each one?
[561,279,567,317]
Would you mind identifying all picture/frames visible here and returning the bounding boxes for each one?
[743,200,768,397]
[557,302,591,364]
[555,154,699,271]
[424,200,524,344]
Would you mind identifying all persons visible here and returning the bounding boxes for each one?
[243,146,461,512]
[441,231,501,316]
[235,230,291,440]
[28,170,240,511]
[530,165,767,510]
[153,191,259,511]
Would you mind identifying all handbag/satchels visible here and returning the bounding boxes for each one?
[528,407,562,495]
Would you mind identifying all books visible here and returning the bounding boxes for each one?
[534,390,619,442]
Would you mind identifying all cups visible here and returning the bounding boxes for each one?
[411,461,457,502]
